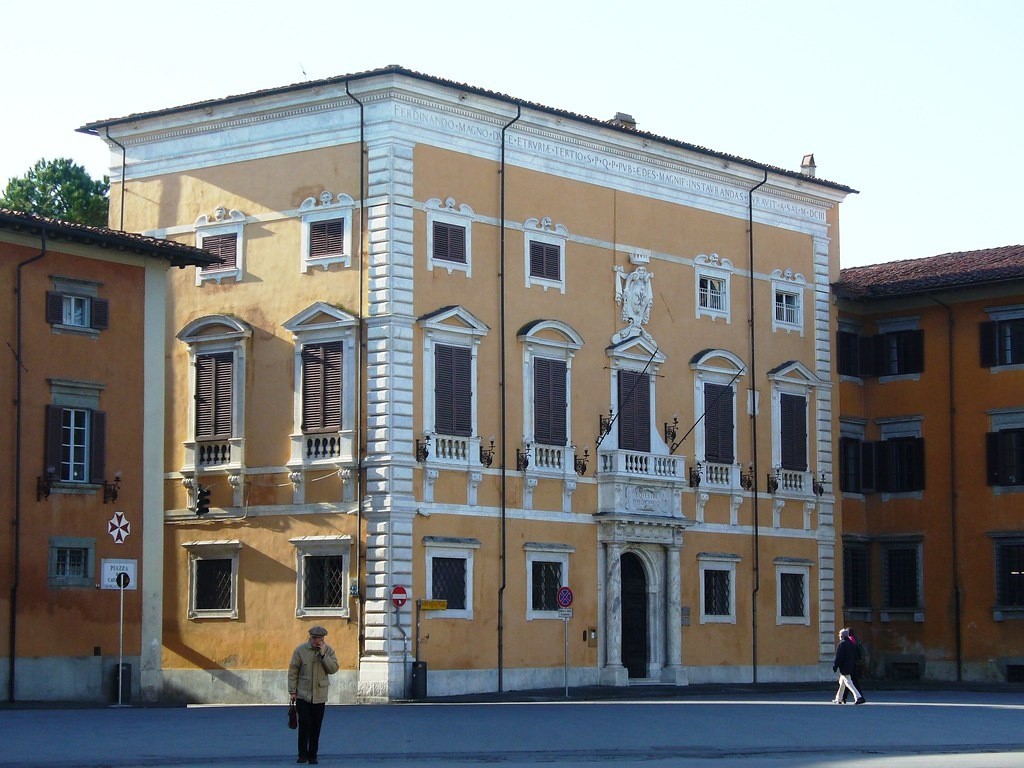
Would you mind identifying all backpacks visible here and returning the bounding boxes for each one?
[854,639,870,666]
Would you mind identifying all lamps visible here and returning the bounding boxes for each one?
[688,460,705,487]
[766,464,782,494]
[813,469,826,497]
[574,445,590,475]
[740,462,754,488]
[416,430,431,463]
[664,412,679,444]
[599,404,614,435]
[516,438,532,472]
[36,466,55,502]
[103,470,122,504]
[479,435,495,468]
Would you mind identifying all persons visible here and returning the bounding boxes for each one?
[288,626,339,765]
[832,627,866,704]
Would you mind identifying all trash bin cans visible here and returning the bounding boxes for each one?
[114,663,132,705]
[408,661,427,701]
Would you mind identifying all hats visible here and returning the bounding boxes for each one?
[308,627,327,637]
[838,629,849,636]
[846,627,853,633]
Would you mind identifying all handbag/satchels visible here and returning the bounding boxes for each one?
[288,705,297,729]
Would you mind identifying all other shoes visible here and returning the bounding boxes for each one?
[855,697,865,704]
[832,700,842,704]
[308,757,318,764]
[842,699,847,703]
[297,759,307,763]
